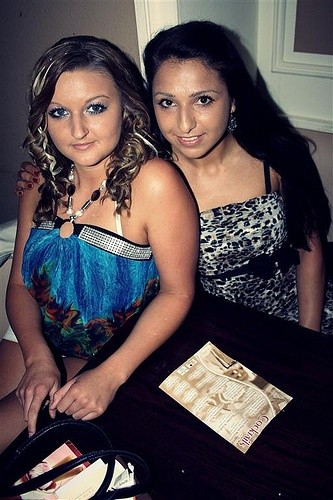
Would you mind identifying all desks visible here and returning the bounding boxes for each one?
[0,283,333,500]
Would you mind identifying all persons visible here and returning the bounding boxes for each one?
[144,21,333,335]
[0,35,201,455]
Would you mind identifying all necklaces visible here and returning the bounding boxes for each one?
[59,162,122,238]
